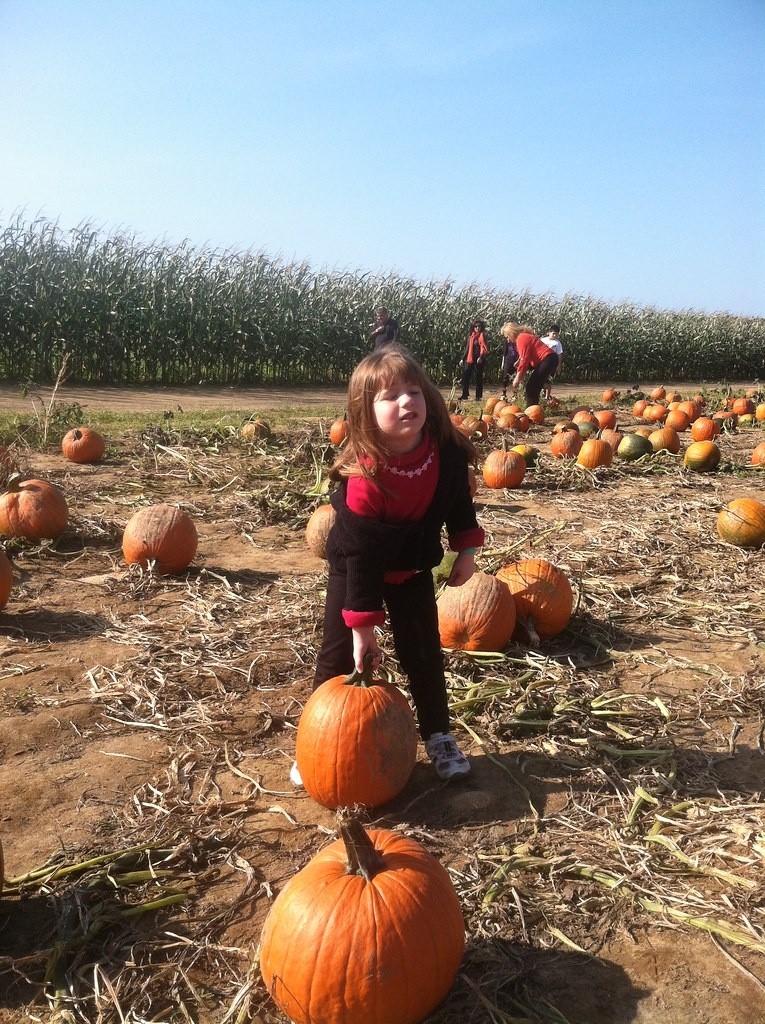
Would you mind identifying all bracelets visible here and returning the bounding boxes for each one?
[460,547,477,554]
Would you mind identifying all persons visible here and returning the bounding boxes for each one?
[369,306,400,353]
[458,320,489,402]
[291,348,488,790]
[500,321,560,409]
[539,325,564,399]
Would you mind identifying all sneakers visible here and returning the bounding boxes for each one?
[289,761,304,789]
[424,734,470,783]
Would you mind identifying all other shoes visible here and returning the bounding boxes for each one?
[475,397,481,401]
[457,395,467,400]
[499,395,507,401]
[510,395,517,402]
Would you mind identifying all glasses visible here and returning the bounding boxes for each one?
[473,325,481,328]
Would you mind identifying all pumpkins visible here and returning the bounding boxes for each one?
[0,547,12,614]
[295,649,415,809]
[240,418,271,435]
[306,389,765,558]
[62,428,105,462]
[437,572,516,652]
[261,806,465,1024]
[0,473,68,540]
[495,559,573,645]
[123,505,197,573]
